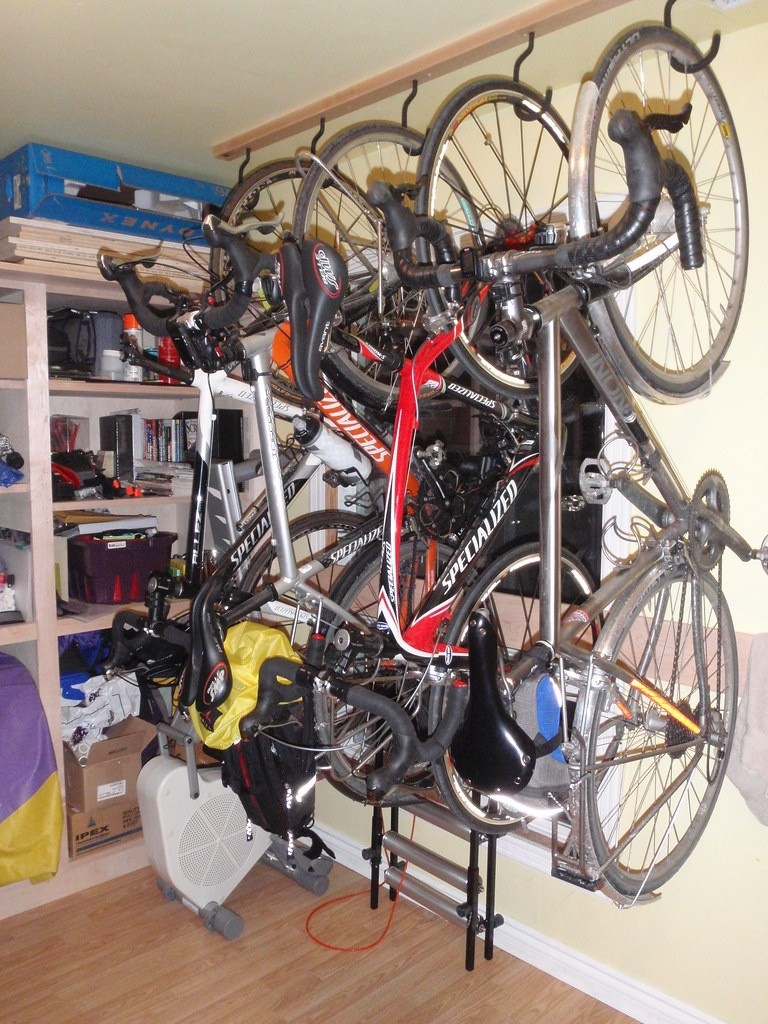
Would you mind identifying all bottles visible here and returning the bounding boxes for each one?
[291,415,374,484]
[99,312,182,386]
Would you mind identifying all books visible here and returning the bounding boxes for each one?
[166,558,186,600]
[133,418,196,496]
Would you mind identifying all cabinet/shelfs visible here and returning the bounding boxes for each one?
[0,261,339,922]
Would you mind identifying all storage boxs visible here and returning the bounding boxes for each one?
[65,801,144,859]
[0,143,236,250]
[63,714,157,811]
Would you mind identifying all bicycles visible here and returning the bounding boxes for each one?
[93,18,768,912]
